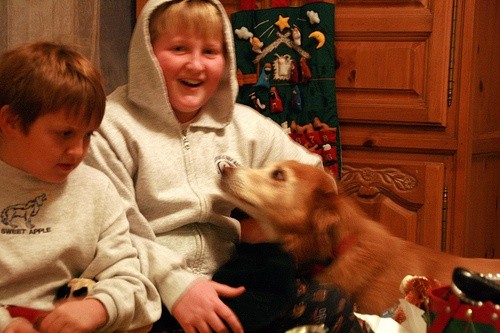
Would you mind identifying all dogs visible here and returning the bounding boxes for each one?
[217,161,500,317]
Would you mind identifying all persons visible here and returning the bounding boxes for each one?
[85,0,336,333]
[0,42,162,333]
